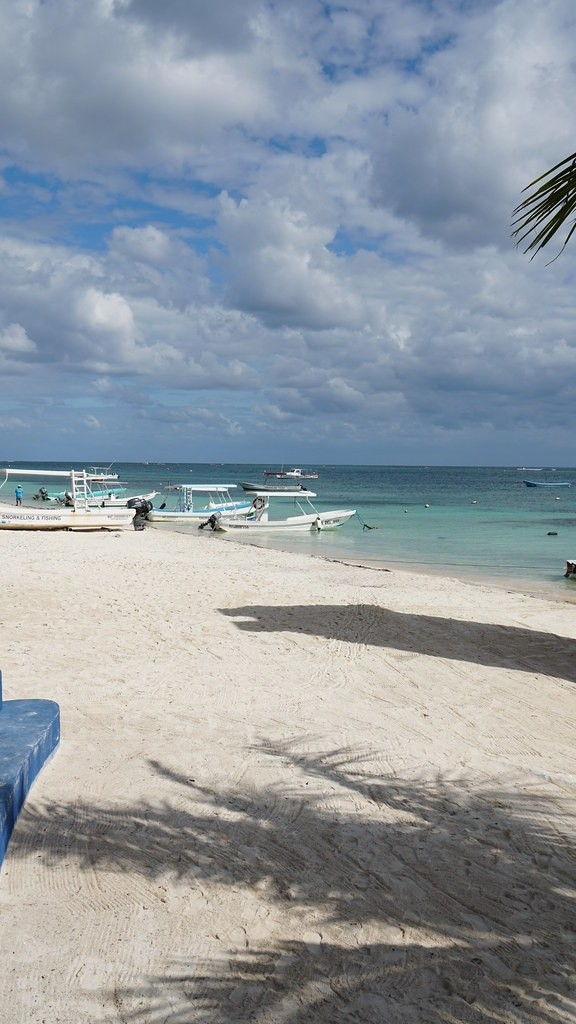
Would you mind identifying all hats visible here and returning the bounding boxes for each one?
[18,485,22,487]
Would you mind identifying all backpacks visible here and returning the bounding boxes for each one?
[16,488,22,497]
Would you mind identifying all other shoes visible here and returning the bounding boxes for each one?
[16,505,18,506]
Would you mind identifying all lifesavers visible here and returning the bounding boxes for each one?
[253,497,265,510]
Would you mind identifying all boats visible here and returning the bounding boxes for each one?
[57,492,159,507]
[144,482,270,522]
[35,480,128,502]
[238,471,304,492]
[276,468,319,480]
[0,467,120,480]
[0,507,137,531]
[210,508,358,533]
[523,480,572,488]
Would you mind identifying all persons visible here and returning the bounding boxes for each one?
[15,485,23,506]
